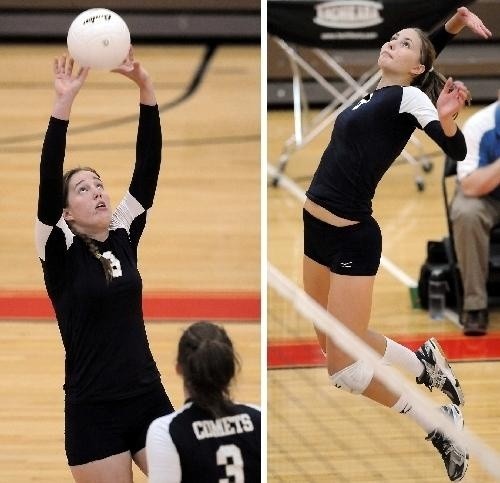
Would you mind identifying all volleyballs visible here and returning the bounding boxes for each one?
[67,7,131,73]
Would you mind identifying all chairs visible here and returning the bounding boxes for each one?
[441,150,499,324]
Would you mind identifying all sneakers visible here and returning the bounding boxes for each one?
[424,404,469,482]
[416,337,465,407]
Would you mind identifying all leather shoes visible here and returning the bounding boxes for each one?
[463,310,488,336]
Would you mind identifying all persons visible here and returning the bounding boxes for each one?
[448,86,500,336]
[33,42,176,483]
[301,5,495,482]
[145,320,261,483]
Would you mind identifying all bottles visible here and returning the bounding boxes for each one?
[428,268,448,320]
